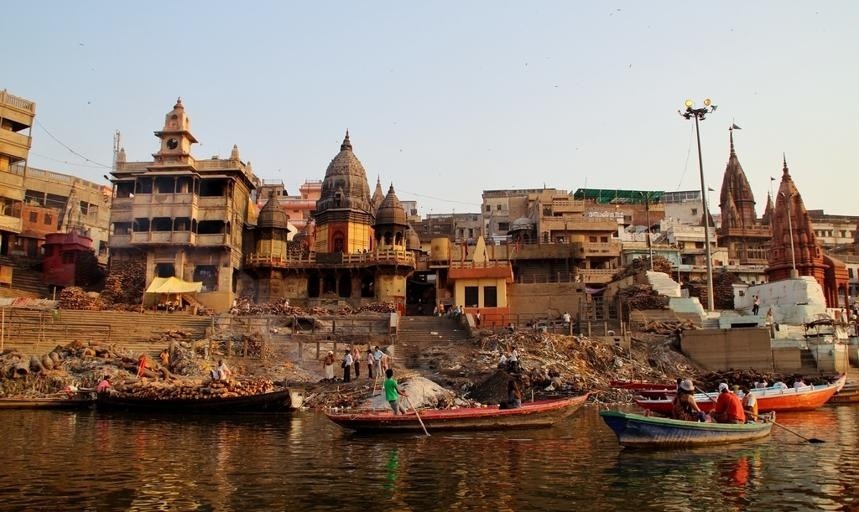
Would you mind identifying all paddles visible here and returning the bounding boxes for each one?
[744,409,825,443]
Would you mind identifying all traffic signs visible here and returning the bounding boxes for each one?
[102,175,115,241]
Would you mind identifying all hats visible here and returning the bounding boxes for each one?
[680,380,694,390]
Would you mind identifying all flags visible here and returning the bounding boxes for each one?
[709,188,714,191]
[733,124,741,130]
[771,177,775,180]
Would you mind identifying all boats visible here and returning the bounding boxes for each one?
[609,431,786,476]
[1,398,98,411]
[637,387,680,399]
[340,428,596,453]
[633,371,850,418]
[95,387,310,415]
[322,389,592,432]
[771,410,840,427]
[598,409,779,448]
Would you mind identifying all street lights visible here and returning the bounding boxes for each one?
[779,191,799,279]
[637,189,665,271]
[676,98,721,310]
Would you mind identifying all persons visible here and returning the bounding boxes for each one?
[208,359,232,383]
[321,345,385,383]
[752,295,761,315]
[498,379,522,409]
[496,352,507,369]
[670,374,807,424]
[159,347,170,369]
[381,368,409,416]
[137,351,147,378]
[432,300,481,326]
[96,375,114,394]
[506,322,514,333]
[765,308,775,325]
[507,346,518,369]
[562,311,571,329]
